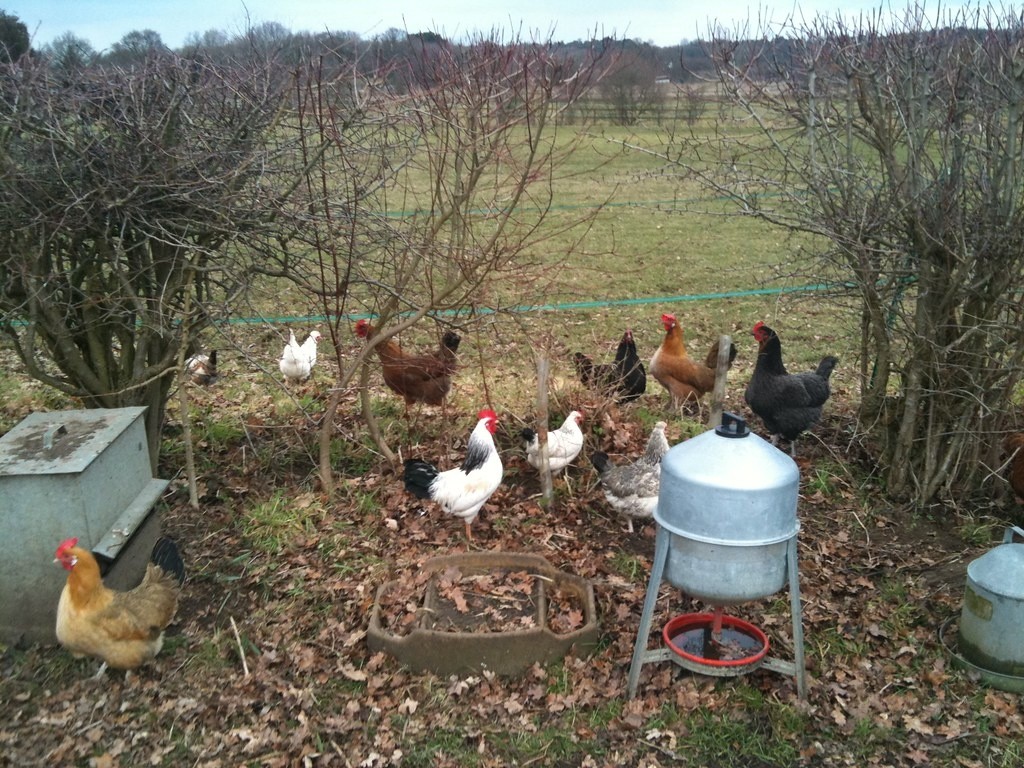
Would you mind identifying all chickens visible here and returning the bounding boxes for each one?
[51,535,189,685]
[180,311,842,543]
